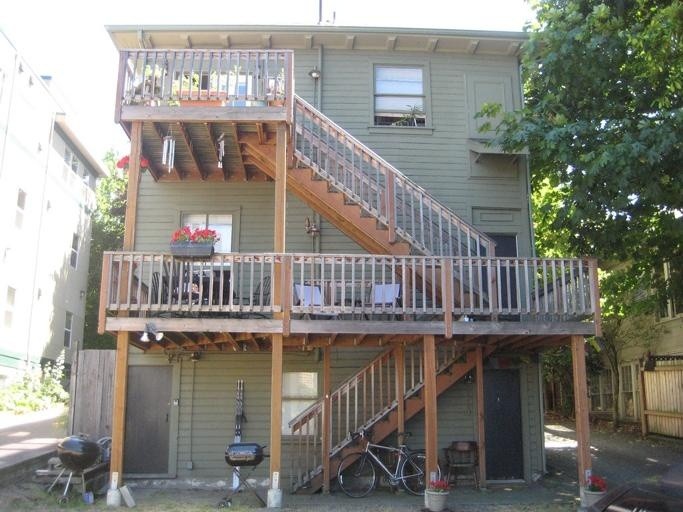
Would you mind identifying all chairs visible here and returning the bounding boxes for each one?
[152,271,400,321]
[443,441,479,491]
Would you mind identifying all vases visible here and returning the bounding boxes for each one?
[583,490,606,508]
[425,489,448,512]
[169,242,214,257]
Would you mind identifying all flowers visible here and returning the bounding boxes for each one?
[172,225,218,242]
[588,475,607,492]
[429,480,451,493]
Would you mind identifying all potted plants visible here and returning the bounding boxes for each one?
[140,65,285,107]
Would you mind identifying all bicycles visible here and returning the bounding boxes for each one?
[333,423,443,498]
